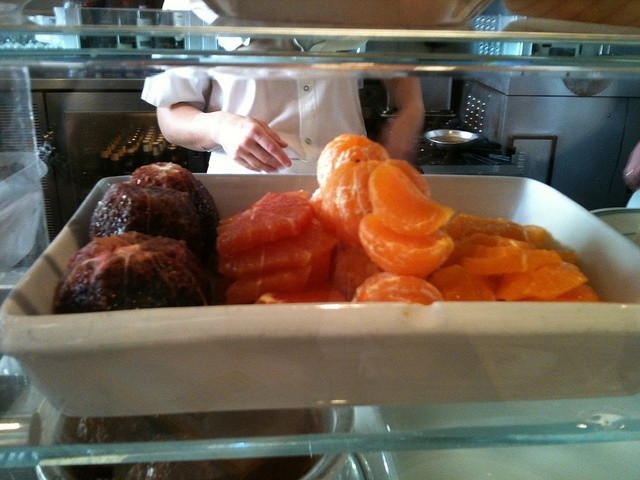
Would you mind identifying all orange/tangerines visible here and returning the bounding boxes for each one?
[310,134,454,304]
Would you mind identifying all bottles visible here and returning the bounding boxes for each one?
[97,126,194,178]
[116,0,135,50]
[155,11,174,49]
[135,0,154,51]
[97,0,116,48]
[172,12,185,49]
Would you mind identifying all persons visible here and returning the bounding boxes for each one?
[624,141,640,208]
[138,1,425,175]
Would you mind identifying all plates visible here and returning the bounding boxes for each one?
[589,206,640,248]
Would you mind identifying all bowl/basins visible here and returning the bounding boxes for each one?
[27,396,356,479]
[424,128,479,150]
